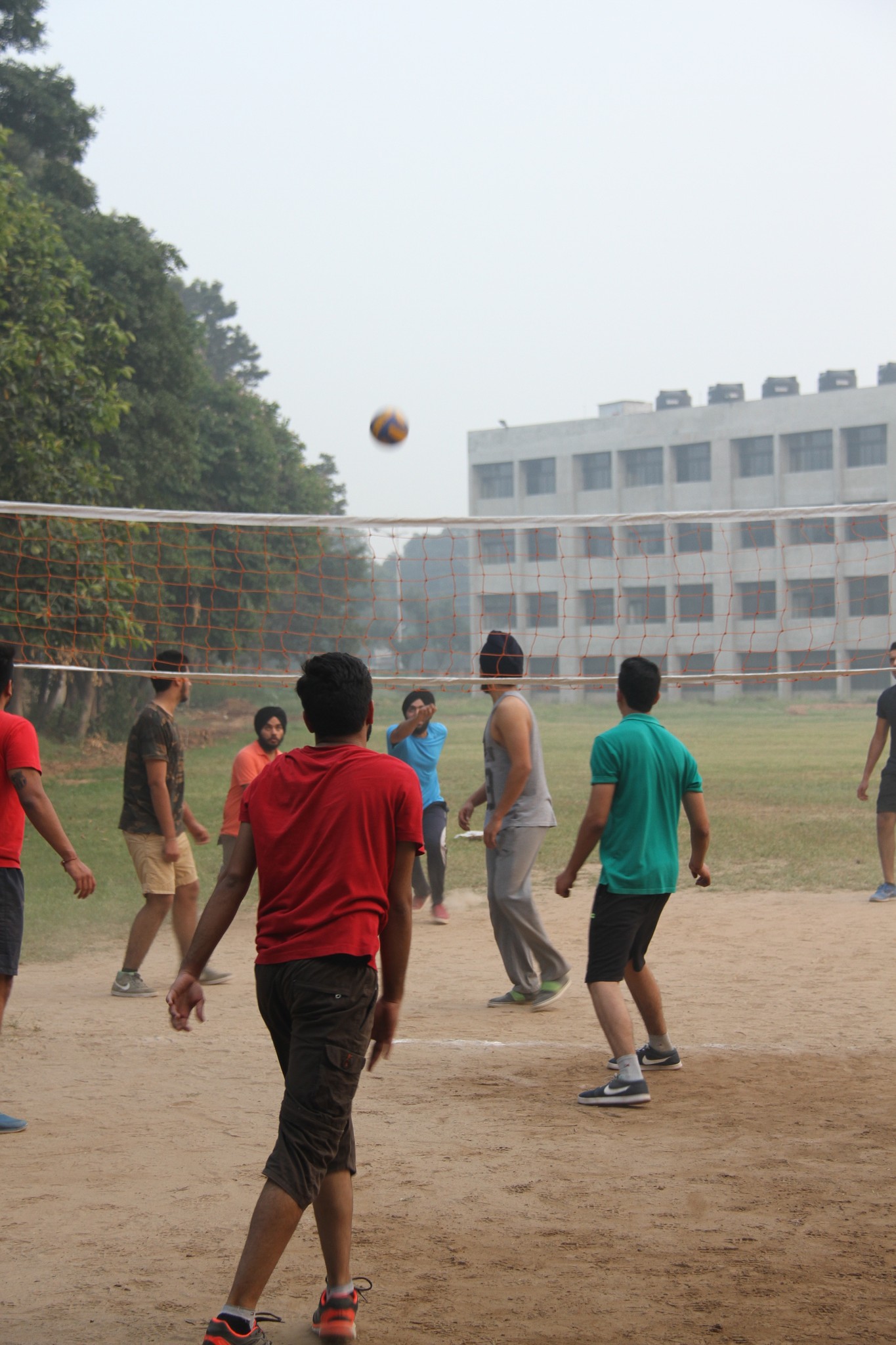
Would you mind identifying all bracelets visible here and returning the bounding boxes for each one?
[61,855,78,866]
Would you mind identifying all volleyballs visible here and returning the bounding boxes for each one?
[369,405,409,443]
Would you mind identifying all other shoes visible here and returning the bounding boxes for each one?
[412,894,431,912]
[869,883,896,902]
[530,974,571,1010]
[487,990,539,1007]
[431,904,449,924]
[0,1112,29,1135]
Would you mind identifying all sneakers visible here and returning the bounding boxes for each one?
[200,967,234,985]
[313,1275,372,1338]
[110,971,160,997]
[202,1312,285,1345]
[608,1042,683,1070]
[577,1072,651,1105]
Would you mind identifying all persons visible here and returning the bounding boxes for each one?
[556,655,713,1104]
[387,690,450,923]
[458,630,572,1010]
[165,652,428,1345]
[111,650,233,996]
[0,644,96,1018]
[857,641,896,901]
[216,707,287,880]
